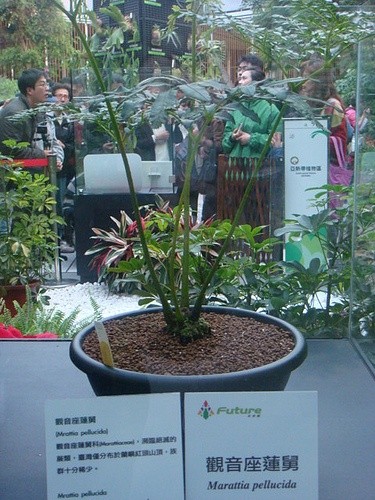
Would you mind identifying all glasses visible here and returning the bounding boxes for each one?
[236,67,248,73]
[58,94,70,100]
[38,83,49,87]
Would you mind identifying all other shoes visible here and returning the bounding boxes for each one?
[60,243,74,253]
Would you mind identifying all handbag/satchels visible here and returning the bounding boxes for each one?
[328,136,353,221]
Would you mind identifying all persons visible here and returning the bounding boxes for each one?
[1,55,369,254]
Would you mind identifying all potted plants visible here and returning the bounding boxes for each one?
[0,137,69,317]
[46,0,311,400]
